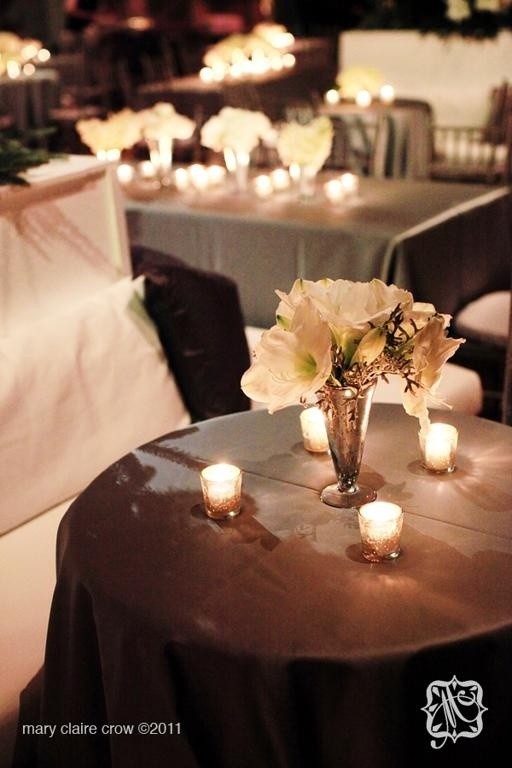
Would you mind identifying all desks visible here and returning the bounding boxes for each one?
[58,397,512,745]
[124,162,509,332]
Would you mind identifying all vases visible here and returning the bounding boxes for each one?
[318,385,379,507]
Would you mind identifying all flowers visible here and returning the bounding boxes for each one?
[240,279,465,430]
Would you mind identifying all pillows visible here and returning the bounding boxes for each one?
[0,275,193,544]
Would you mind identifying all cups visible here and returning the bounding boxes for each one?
[199,463,243,521]
[417,422,460,474]
[299,406,330,453]
[358,500,405,563]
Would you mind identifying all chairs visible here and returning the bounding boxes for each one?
[0,168,134,739]
